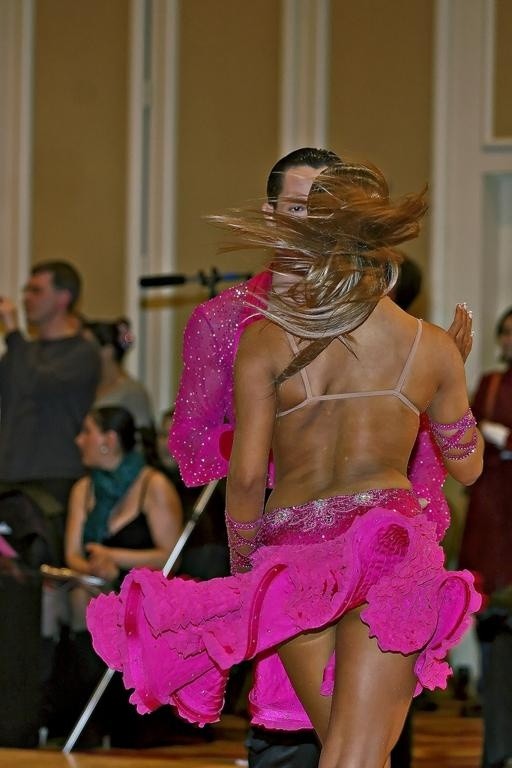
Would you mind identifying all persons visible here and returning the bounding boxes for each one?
[1,405,258,751]
[0,260,101,567]
[85,320,155,460]
[167,146,411,768]
[416,504,485,715]
[85,159,489,768]
[463,307,512,768]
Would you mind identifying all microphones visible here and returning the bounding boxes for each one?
[140,276,185,287]
